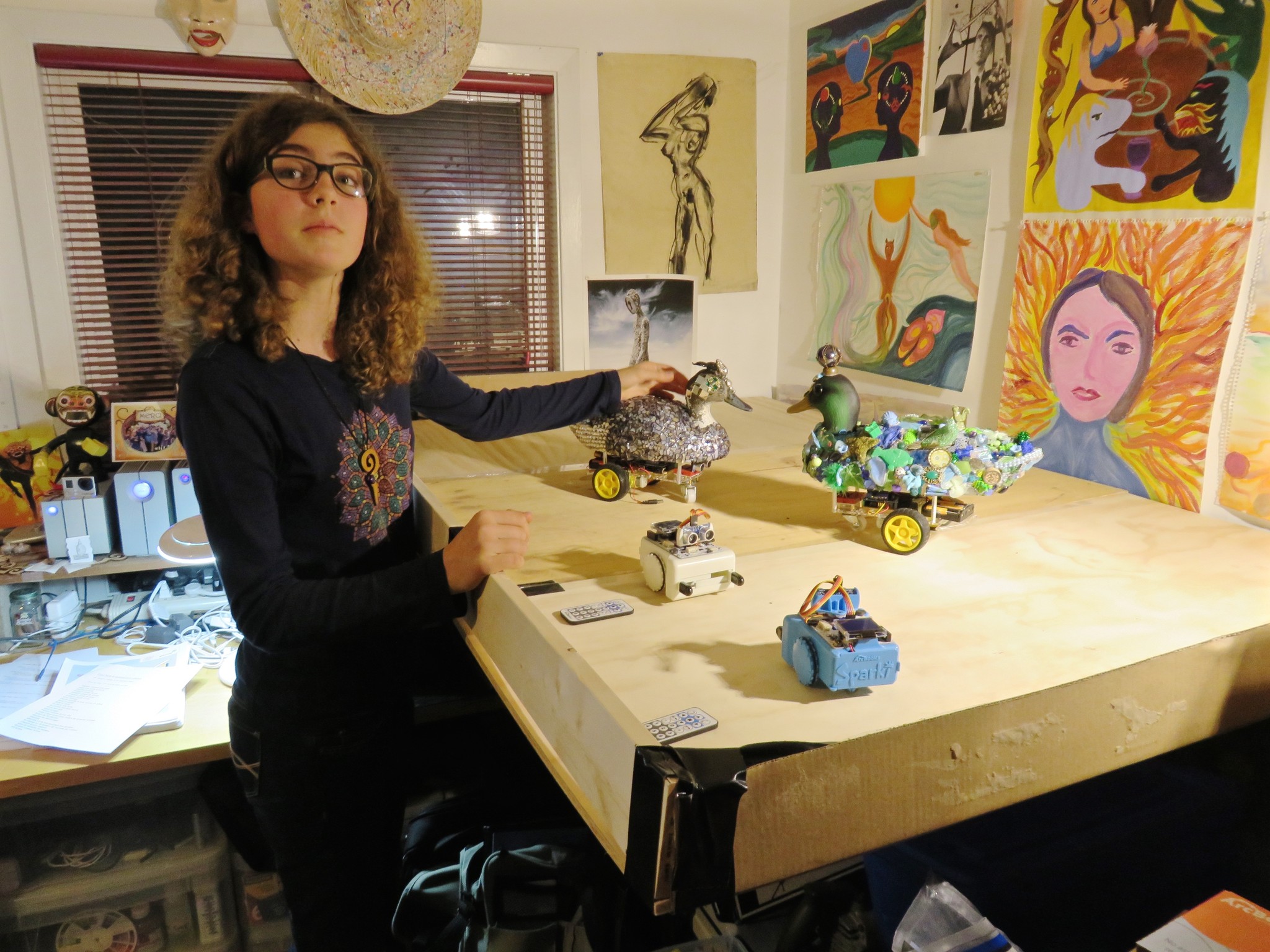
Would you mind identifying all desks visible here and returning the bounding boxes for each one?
[0,516,248,808]
[397,367,1269,952]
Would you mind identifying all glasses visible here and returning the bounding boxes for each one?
[238,153,373,199]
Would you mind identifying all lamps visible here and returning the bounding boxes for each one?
[158,512,218,566]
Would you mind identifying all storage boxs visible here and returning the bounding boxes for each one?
[1,786,243,952]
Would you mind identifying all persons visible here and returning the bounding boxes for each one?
[169,0,238,56]
[120,413,180,453]
[147,90,688,952]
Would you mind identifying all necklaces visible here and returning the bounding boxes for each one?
[273,319,379,507]
